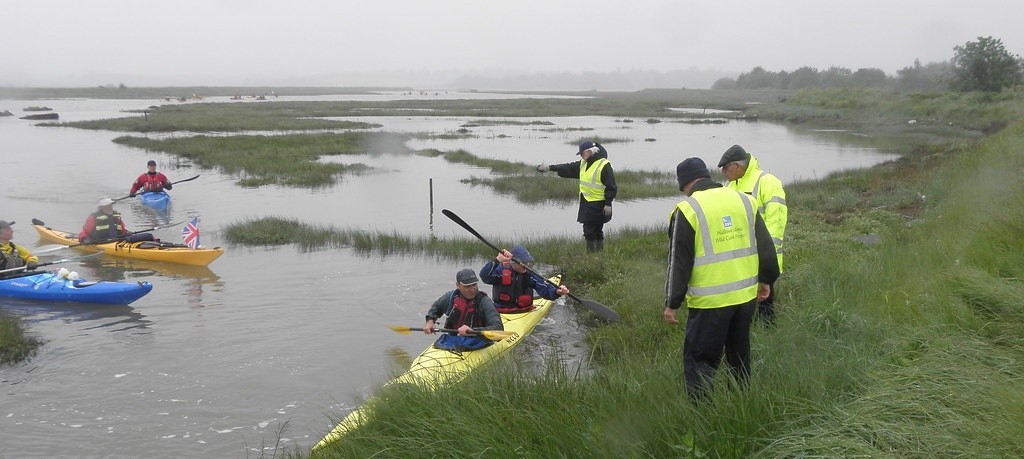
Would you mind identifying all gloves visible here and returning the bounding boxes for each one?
[27,261,37,270]
[603,205,612,219]
[164,181,171,189]
[537,164,550,173]
[129,192,136,197]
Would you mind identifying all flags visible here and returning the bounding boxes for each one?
[182,216,200,249]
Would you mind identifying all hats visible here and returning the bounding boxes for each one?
[95,198,115,207]
[511,246,535,263]
[678,158,710,190]
[718,145,747,167]
[576,141,596,155]
[456,268,479,286]
[0,220,15,228]
[148,161,157,166]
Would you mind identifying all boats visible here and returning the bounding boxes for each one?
[307,274,562,459]
[30,219,224,267]
[247,96,267,100]
[230,97,245,100]
[0,270,153,306]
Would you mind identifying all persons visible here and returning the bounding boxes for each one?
[716,145,788,328]
[78,198,130,243]
[536,141,617,255]
[0,221,39,279]
[479,247,569,313]
[424,269,504,351]
[663,157,781,407]
[129,160,172,197]
